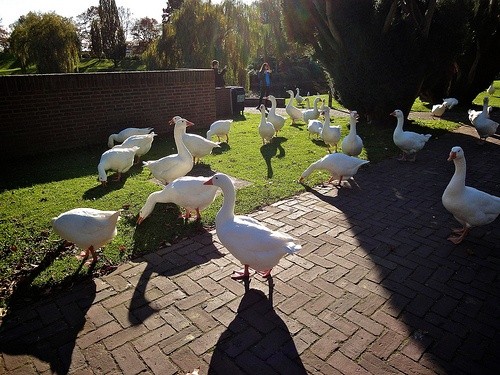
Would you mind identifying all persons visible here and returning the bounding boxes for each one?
[255,63,272,113]
[211,60,228,88]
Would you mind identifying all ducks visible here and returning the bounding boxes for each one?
[47,87,500,283]
